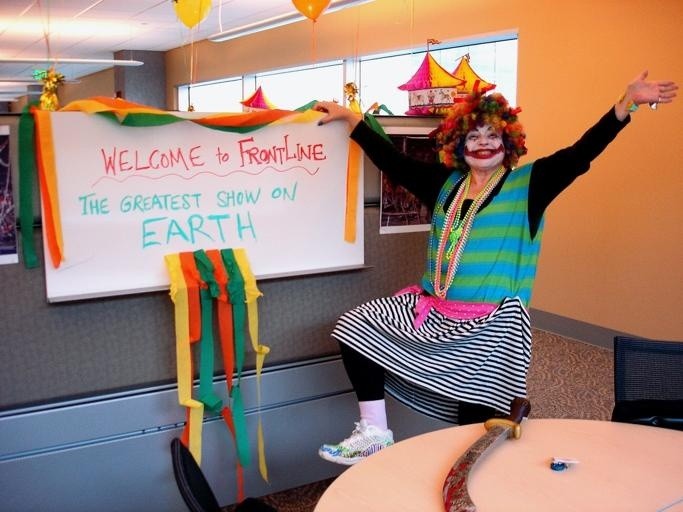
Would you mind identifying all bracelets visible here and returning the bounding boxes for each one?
[618,90,640,113]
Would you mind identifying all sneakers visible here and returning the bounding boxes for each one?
[318,419,395,467]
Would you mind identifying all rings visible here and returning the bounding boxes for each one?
[656,96,663,104]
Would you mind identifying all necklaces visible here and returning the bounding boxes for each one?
[427,163,507,302]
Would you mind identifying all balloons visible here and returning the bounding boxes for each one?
[171,0,212,29]
[291,0,333,21]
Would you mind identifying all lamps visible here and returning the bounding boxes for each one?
[0,20,145,104]
[206,0,375,44]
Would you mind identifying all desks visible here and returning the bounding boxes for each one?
[307,418,682,512]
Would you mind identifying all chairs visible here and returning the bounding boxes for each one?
[607,332,683,433]
[168,435,287,512]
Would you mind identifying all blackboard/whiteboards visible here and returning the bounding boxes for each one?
[40,111,379,306]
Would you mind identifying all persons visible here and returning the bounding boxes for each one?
[309,70,680,467]
